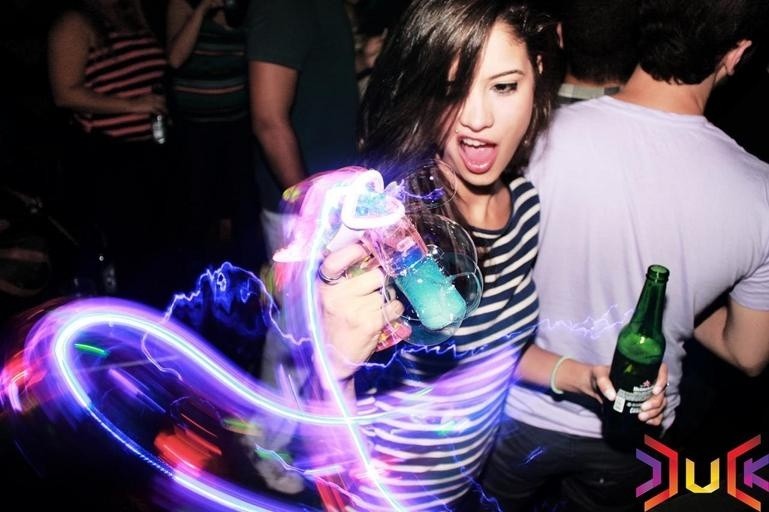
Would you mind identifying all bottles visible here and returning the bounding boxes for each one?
[153,82,169,146]
[605,264,668,458]
